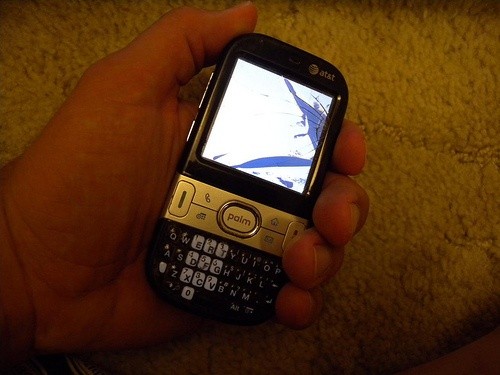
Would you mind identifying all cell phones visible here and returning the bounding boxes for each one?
[144,33,348,326]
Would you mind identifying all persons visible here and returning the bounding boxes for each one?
[0,0,372,371]
